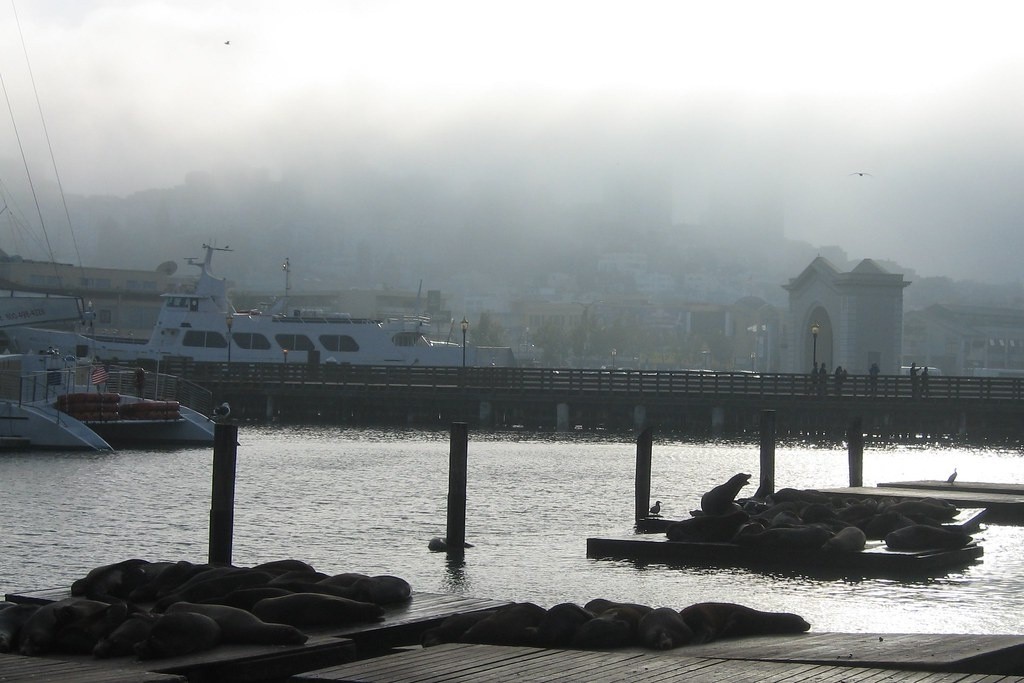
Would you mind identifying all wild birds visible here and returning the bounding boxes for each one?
[944,468,957,485]
[650,501,662,519]
[207,402,230,424]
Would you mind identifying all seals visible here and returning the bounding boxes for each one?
[428,536,472,552]
[418,600,812,649]
[0,558,411,655]
[666,473,972,551]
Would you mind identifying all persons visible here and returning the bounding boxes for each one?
[910,361,930,398]
[870,362,880,396]
[811,358,848,396]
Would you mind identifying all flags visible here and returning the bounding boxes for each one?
[91,365,111,385]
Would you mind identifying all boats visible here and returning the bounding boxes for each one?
[77,239,521,368]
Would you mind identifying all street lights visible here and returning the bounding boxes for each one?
[810,324,820,374]
[460,315,469,366]
[226,312,234,382]
[282,348,288,363]
[571,300,605,352]
[610,348,617,370]
